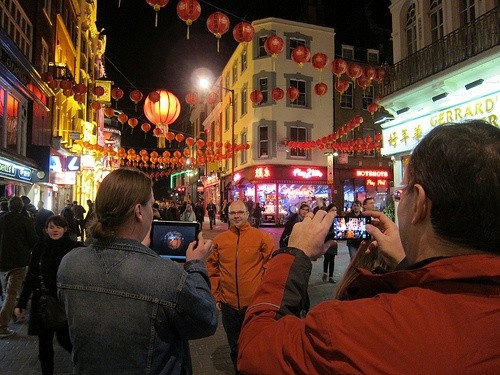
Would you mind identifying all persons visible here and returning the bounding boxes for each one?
[322,204,338,283]
[253,202,265,229]
[221,199,253,223]
[236,120,500,375]
[206,199,217,230]
[15,216,85,374]
[152,198,205,230]
[346,196,375,262]
[206,200,276,372]
[57,168,219,375]
[312,197,326,215]
[347,229,354,238]
[0,195,94,243]
[168,232,183,249]
[279,204,310,247]
[0,196,37,336]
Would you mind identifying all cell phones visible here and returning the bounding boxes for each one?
[328,215,371,239]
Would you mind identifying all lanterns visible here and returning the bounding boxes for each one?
[283,114,382,153]
[146,1,385,106]
[185,91,200,105]
[143,87,181,148]
[165,133,251,152]
[314,82,328,104]
[206,92,219,105]
[76,141,232,181]
[250,89,263,105]
[367,103,378,115]
[43,70,163,137]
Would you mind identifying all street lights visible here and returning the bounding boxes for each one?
[199,78,234,201]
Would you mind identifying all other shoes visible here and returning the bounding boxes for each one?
[329,278,336,283]
[322,274,327,281]
[0,330,14,337]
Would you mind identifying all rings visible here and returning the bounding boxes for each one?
[305,215,312,220]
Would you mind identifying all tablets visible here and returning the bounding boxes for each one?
[149,219,201,263]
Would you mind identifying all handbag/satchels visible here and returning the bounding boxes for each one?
[37,295,66,324]
